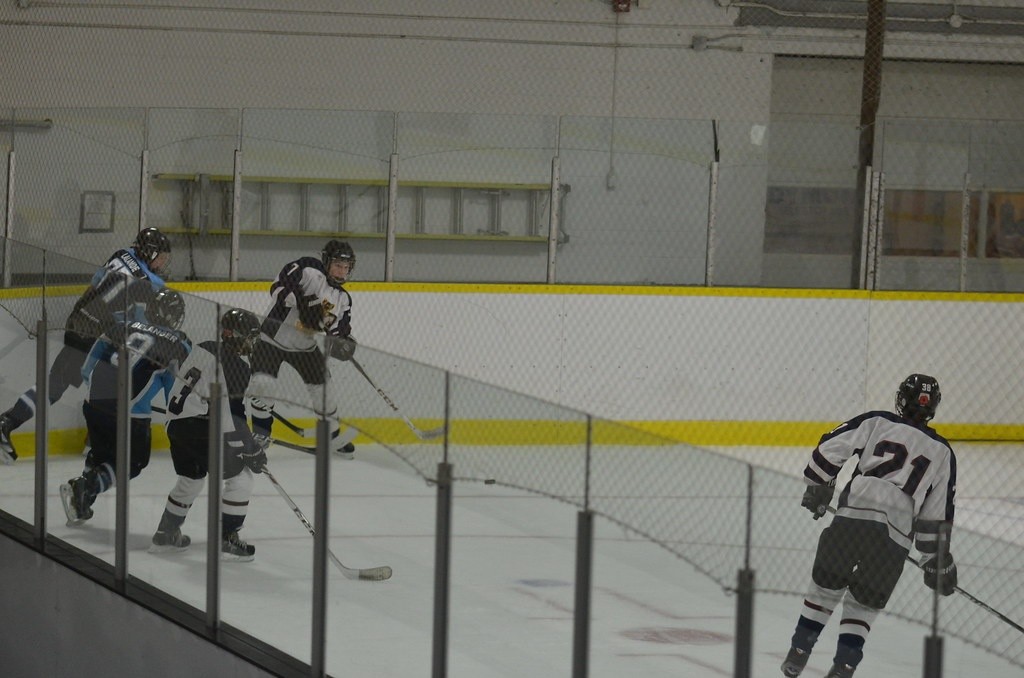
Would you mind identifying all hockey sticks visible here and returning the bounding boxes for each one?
[268,409,341,442]
[260,462,393,582]
[152,404,362,456]
[322,324,447,441]
[828,505,1024,633]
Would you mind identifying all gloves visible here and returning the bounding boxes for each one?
[299,295,324,332]
[924,553,957,596]
[801,486,835,520]
[243,444,268,474]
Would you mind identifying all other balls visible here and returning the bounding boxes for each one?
[484,479,495,484]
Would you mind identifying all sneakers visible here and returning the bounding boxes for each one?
[252,433,271,450]
[147,526,191,555]
[59,471,100,528]
[0,413,24,465]
[222,525,255,562]
[331,429,355,460]
[825,662,855,678]
[781,646,810,678]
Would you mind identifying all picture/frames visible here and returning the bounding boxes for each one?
[80,190,114,233]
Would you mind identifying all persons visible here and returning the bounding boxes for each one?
[60,287,193,524]
[0,227,172,463]
[245,241,357,461]
[148,307,268,562]
[780,372,957,678]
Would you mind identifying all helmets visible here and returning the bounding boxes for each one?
[144,287,186,325]
[322,240,356,273]
[136,227,171,263]
[897,374,941,421]
[222,307,261,338]
